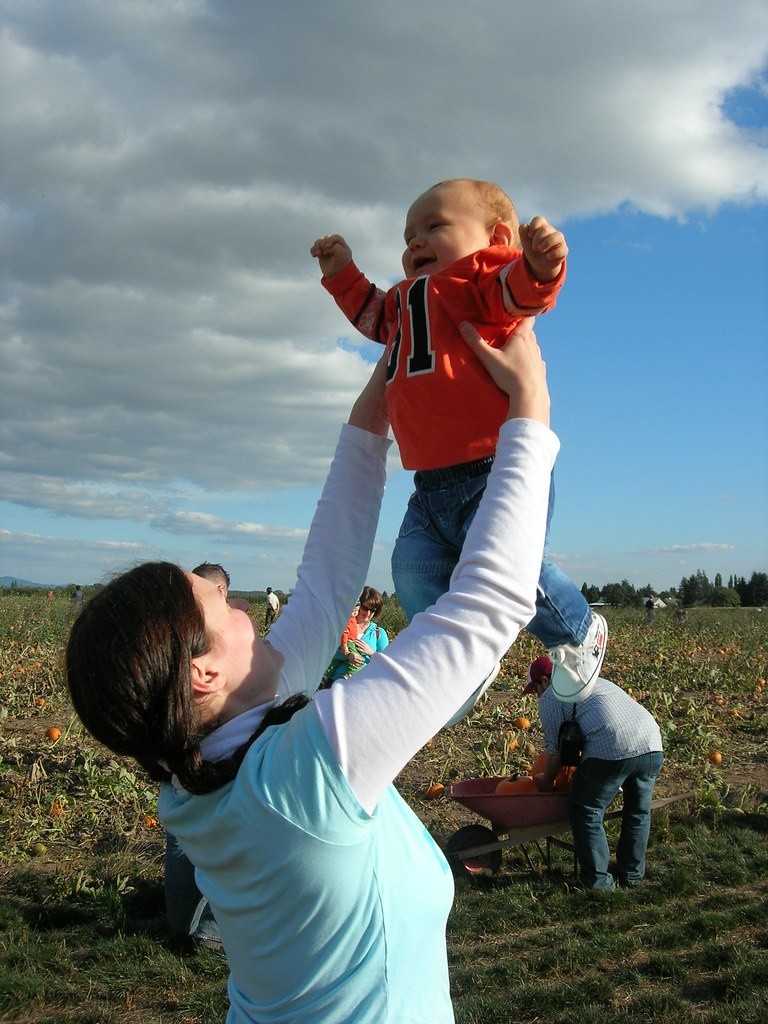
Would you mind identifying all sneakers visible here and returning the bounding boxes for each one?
[549,612,608,703]
[443,662,501,726]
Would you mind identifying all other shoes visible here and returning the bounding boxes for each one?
[342,673,349,680]
[318,677,325,690]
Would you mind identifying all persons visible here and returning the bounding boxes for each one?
[311,181,614,700]
[72,585,83,604]
[66,319,562,1024]
[47,591,55,602]
[319,586,390,690]
[520,654,663,888]
[263,587,280,627]
[166,560,225,955]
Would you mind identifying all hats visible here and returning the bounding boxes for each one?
[522,654,552,696]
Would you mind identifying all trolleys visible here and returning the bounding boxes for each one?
[444,765,695,892]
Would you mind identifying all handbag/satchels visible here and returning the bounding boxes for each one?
[557,704,586,757]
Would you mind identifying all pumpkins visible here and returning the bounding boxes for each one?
[32,843,46,857]
[142,815,156,827]
[45,726,61,741]
[424,648,764,799]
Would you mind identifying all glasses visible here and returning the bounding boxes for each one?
[362,604,375,613]
